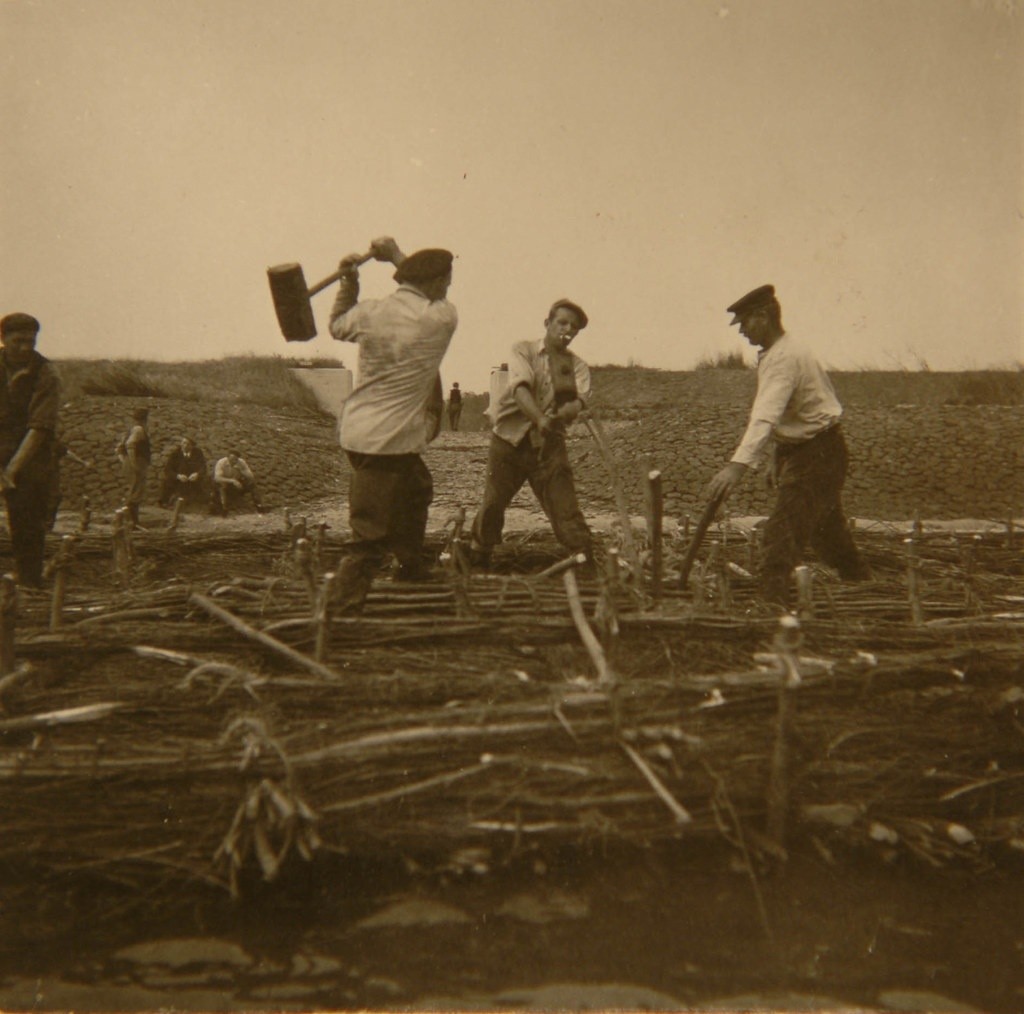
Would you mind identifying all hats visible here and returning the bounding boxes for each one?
[552,299,587,328]
[0,312,39,336]
[392,248,453,282]
[183,431,198,443]
[727,284,774,325]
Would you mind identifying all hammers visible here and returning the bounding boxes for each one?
[266,246,375,342]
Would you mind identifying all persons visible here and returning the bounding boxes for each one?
[328,237,457,617]
[115,408,151,525]
[0,312,60,590]
[707,284,869,582]
[445,382,463,431]
[214,449,265,517]
[158,436,208,509]
[470,299,597,582]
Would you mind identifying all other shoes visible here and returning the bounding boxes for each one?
[221,508,228,516]
[257,503,271,511]
